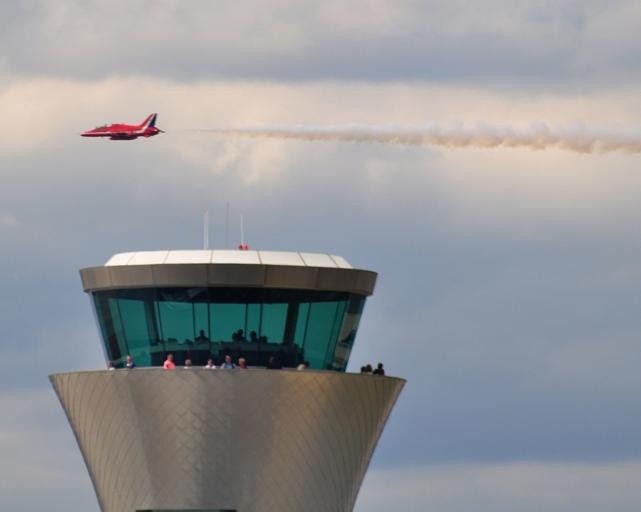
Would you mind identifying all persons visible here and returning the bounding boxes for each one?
[250,330,258,343]
[367,364,373,374]
[164,353,176,368]
[239,357,247,369]
[297,361,310,369]
[361,366,367,374]
[206,358,217,369]
[196,329,209,343]
[221,354,237,368]
[373,362,385,375]
[125,355,136,368]
[107,359,116,370]
[184,358,192,369]
[260,335,268,343]
[234,328,245,341]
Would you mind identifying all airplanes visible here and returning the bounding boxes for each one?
[80,112,165,140]
